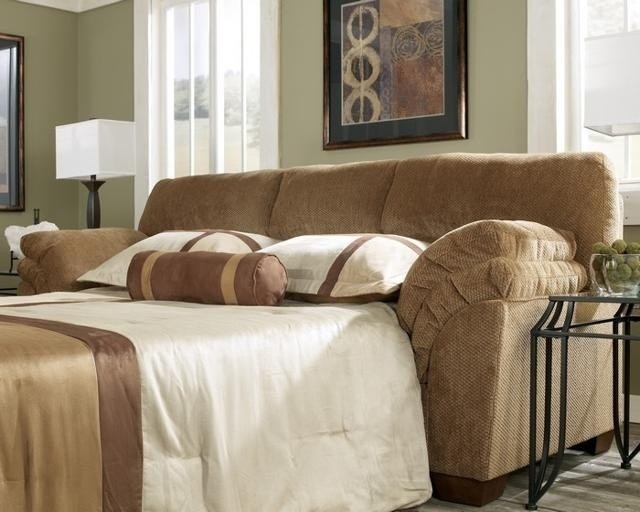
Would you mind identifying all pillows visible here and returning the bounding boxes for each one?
[254,233,430,305]
[121,249,289,305]
[73,225,282,287]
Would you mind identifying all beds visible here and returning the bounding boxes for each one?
[0,152,629,512]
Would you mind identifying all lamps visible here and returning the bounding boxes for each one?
[51,115,138,228]
[579,29,640,139]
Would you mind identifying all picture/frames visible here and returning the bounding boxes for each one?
[1,32,27,213]
[320,0,469,148]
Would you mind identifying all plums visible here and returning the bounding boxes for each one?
[592,240,640,292]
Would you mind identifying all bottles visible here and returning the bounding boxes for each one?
[32,208,41,224]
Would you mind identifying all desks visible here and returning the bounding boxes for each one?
[0,249,24,297]
[524,291,640,510]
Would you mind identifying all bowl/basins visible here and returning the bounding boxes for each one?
[588,254,640,298]
